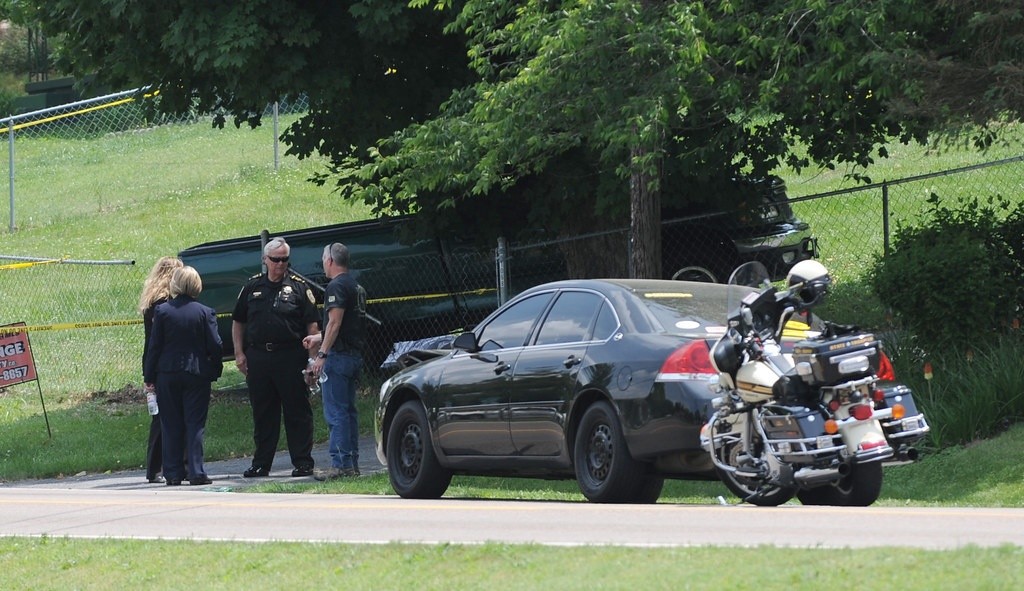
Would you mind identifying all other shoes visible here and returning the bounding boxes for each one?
[149,475,166,482]
[182,471,190,480]
[190,477,212,485]
[165,479,181,485]
[314,467,361,481]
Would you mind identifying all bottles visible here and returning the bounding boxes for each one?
[307,358,328,384]
[301,369,320,395]
[146,388,159,416]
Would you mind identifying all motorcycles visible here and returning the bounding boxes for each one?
[697,237,932,508]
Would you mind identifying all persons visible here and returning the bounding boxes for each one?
[144,266,224,485]
[303,243,367,480]
[140,257,184,483]
[231,238,322,477]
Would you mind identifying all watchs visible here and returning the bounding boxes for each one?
[317,352,327,359]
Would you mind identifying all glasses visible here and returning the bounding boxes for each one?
[266,255,290,262]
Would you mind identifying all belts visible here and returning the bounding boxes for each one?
[251,342,300,352]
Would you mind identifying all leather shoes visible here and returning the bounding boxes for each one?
[244,466,269,477]
[292,465,313,477]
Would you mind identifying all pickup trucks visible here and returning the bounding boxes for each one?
[177,133,824,368]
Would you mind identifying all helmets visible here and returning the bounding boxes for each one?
[787,259,830,307]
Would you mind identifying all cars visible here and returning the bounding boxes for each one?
[372,277,828,505]
